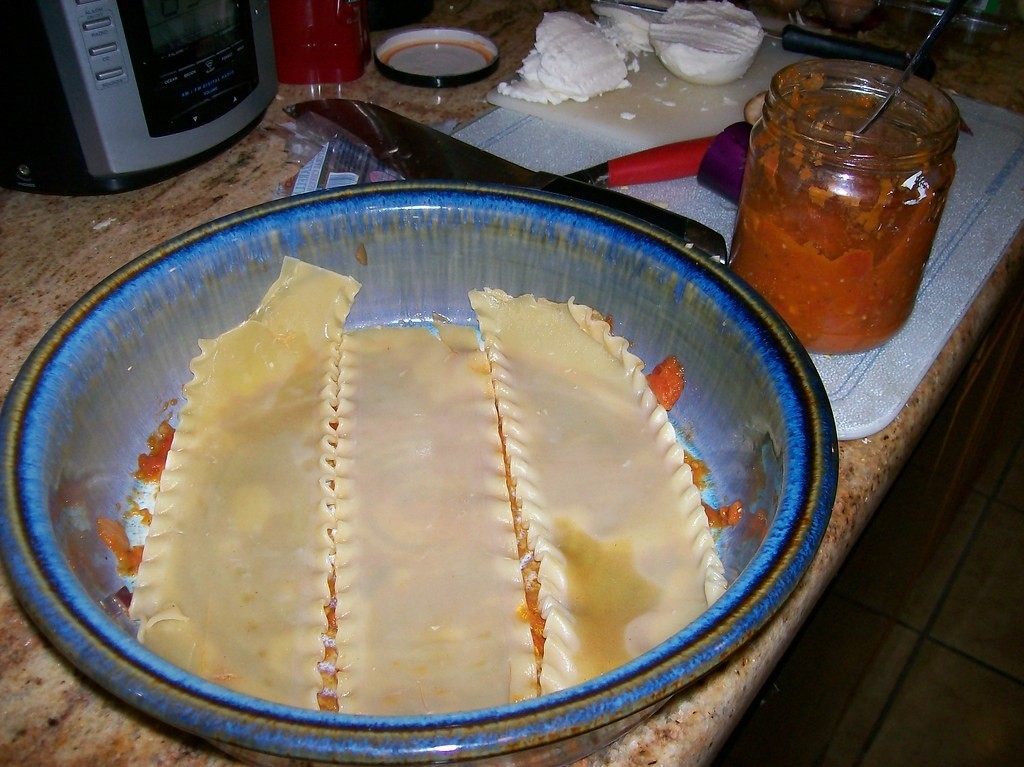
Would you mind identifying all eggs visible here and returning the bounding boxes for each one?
[770,0,877,30]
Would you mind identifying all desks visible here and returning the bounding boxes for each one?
[0,0,1024,767]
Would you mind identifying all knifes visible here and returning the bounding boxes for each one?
[593,0,913,70]
[280,98,728,268]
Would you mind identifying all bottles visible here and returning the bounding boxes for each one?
[747,0,1016,40]
[267,0,435,84]
[728,58,961,354]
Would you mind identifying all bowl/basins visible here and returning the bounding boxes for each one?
[0,183,842,767]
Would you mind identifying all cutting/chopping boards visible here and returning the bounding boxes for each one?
[487,12,816,155]
